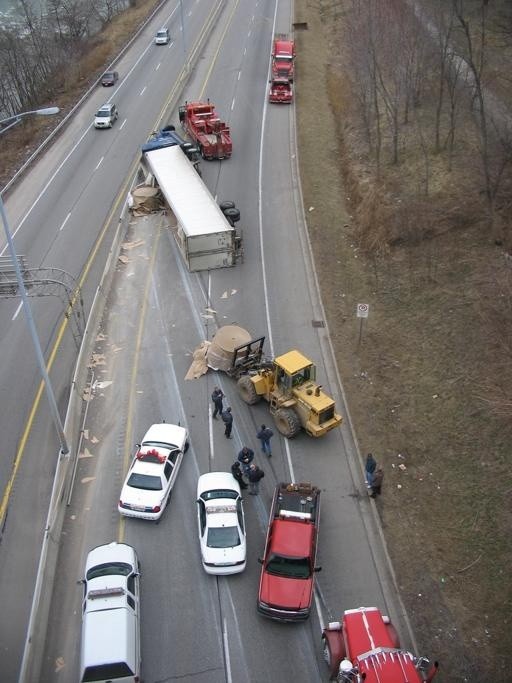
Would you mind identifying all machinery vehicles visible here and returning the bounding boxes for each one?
[200,328,342,443]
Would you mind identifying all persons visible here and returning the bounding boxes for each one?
[222,406,233,439]
[231,461,248,489]
[364,452,377,489]
[256,423,274,457]
[238,446,255,474]
[211,383,226,420]
[368,464,385,498]
[244,463,265,496]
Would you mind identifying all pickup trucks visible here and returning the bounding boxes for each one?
[269,76,293,105]
[80,540,146,683]
[257,481,321,628]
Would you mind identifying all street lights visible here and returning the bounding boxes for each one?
[1,106,73,458]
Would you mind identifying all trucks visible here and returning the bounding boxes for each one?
[144,124,243,277]
[270,31,295,79]
[177,98,234,161]
[319,604,439,683]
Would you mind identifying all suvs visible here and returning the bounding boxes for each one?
[156,27,171,45]
[93,103,119,130]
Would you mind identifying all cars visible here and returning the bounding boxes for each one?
[194,469,249,576]
[99,71,119,89]
[114,417,189,525]
